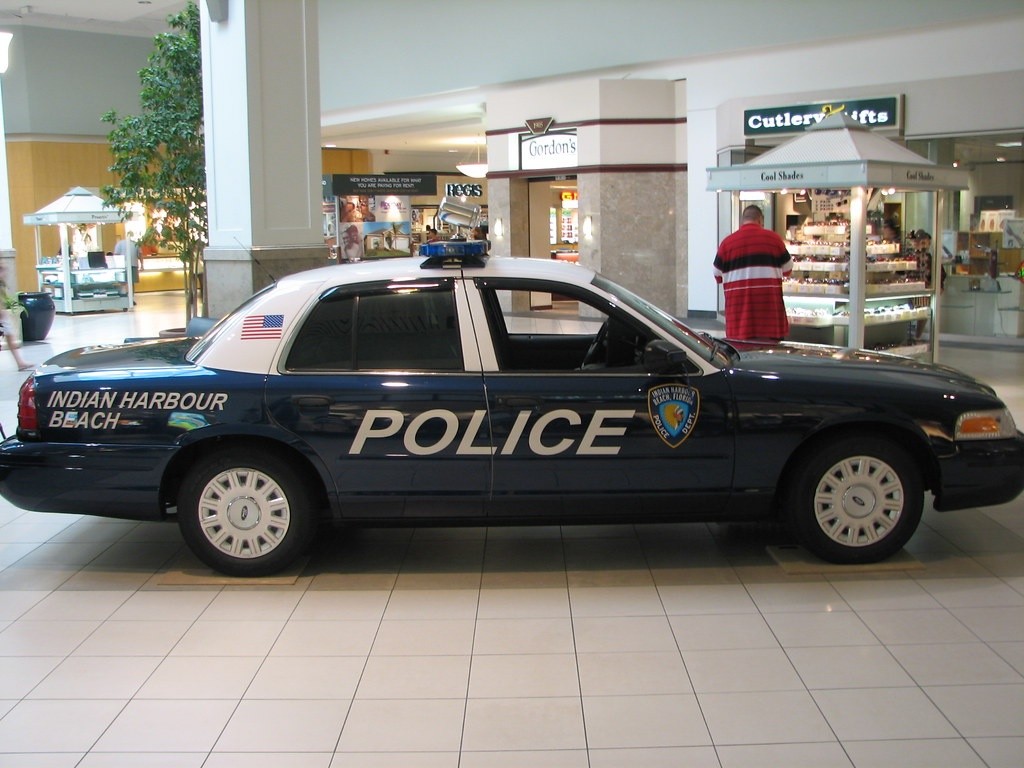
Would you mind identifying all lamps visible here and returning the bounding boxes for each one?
[456,131,488,179]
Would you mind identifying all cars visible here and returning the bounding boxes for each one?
[0,196,1023,578]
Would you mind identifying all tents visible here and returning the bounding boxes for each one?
[705,110,972,363]
[23,186,139,315]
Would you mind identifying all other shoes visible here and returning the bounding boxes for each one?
[18,361,35,371]
[133,301,136,305]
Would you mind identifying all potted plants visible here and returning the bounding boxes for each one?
[99,0,209,337]
[0,289,28,350]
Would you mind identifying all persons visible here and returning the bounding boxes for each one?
[188,240,204,304]
[0,260,37,370]
[712,204,795,341]
[340,194,375,258]
[897,229,947,342]
[113,231,144,305]
[74,223,95,256]
[424,223,491,252]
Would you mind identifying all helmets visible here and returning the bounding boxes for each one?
[359,195,368,205]
